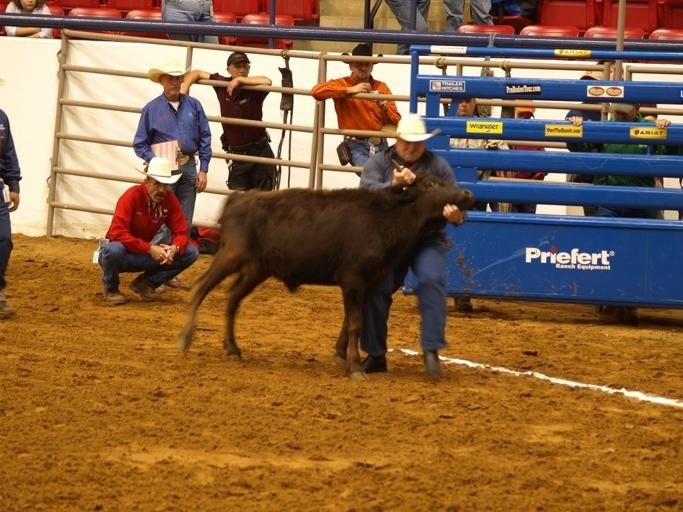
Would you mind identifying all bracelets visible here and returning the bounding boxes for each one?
[175,244,179,253]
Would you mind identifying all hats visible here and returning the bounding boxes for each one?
[580,71,602,80]
[394,114,441,142]
[342,41,384,64]
[147,59,195,83]
[226,51,250,66]
[515,106,538,119]
[134,156,184,185]
[610,103,641,114]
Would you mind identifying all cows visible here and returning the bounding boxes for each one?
[163,158,476,384]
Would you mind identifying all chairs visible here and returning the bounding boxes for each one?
[0,0,681,55]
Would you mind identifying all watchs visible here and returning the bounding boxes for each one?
[452,216,465,225]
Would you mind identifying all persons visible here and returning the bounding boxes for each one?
[678,177,683,220]
[386,0,432,55]
[4,0,54,39]
[92,157,199,303]
[439,0,494,56]
[360,112,469,380]
[181,50,278,191]
[564,69,609,217]
[0,109,21,320]
[450,97,499,212]
[474,104,499,212]
[162,0,219,44]
[568,102,672,220]
[133,59,211,291]
[496,106,548,214]
[312,42,401,177]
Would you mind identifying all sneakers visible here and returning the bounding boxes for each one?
[129,281,162,302]
[599,305,641,322]
[165,277,186,290]
[361,355,388,373]
[422,351,442,377]
[455,298,475,313]
[101,290,127,304]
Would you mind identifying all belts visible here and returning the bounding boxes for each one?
[217,134,269,151]
